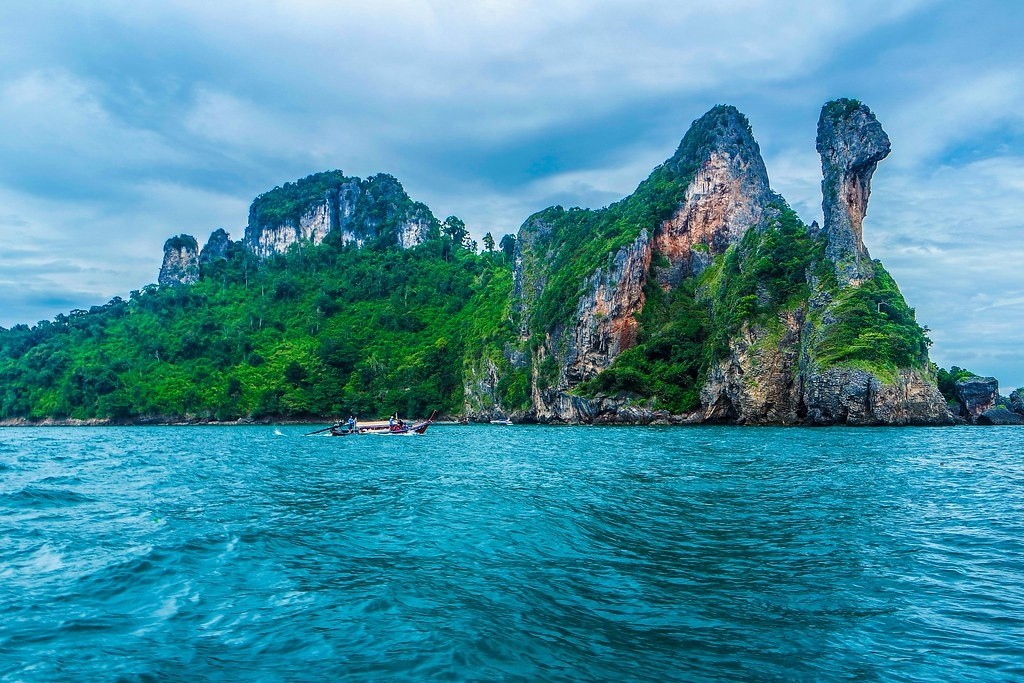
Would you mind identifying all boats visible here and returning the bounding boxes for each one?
[490,419,514,426]
[329,420,430,437]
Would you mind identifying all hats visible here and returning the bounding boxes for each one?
[391,416,394,418]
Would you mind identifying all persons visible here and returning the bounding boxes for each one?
[389,416,408,431]
[348,416,354,433]
[335,419,343,425]
